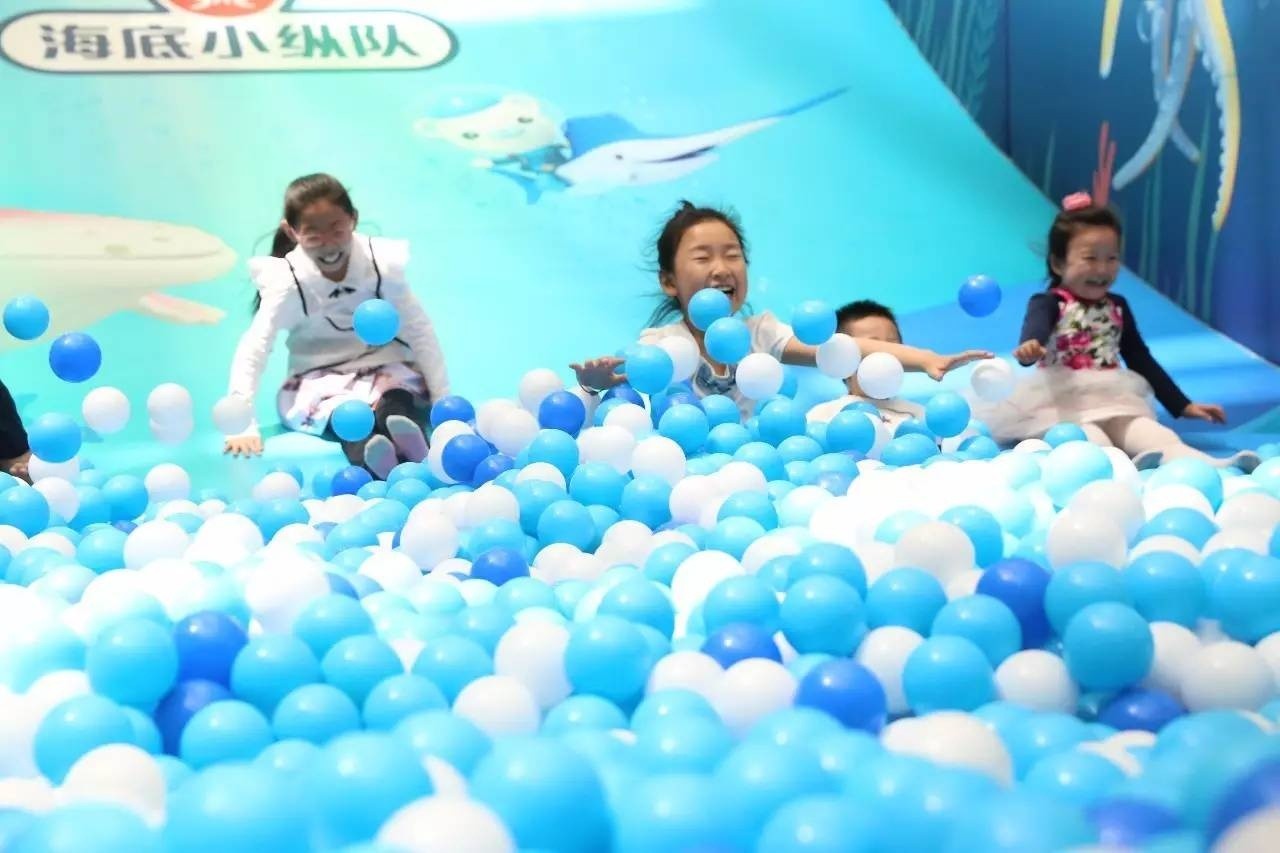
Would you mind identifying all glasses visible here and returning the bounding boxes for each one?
[288,220,353,248]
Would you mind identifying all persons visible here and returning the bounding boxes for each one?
[221,172,449,481]
[570,199,994,421]
[805,299,923,435]
[0,384,33,488]
[1014,193,1261,474]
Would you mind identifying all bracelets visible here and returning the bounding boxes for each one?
[581,385,604,395]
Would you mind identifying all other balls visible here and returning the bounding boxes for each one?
[352,300,401,348]
[6,294,48,339]
[958,275,1003,318]
[0,289,1280,853]
[51,335,99,382]
